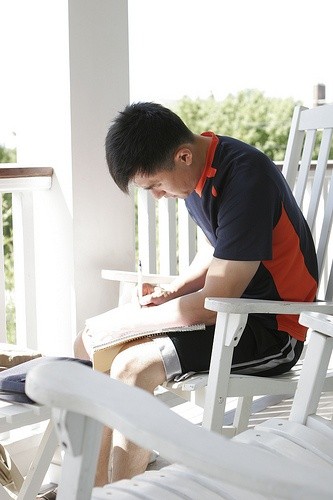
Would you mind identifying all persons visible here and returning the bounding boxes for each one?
[73,102,319,485]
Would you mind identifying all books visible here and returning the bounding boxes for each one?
[84,285,205,353]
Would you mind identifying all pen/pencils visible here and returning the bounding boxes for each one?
[138,261,144,309]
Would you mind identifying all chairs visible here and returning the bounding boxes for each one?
[0,103,333,500]
